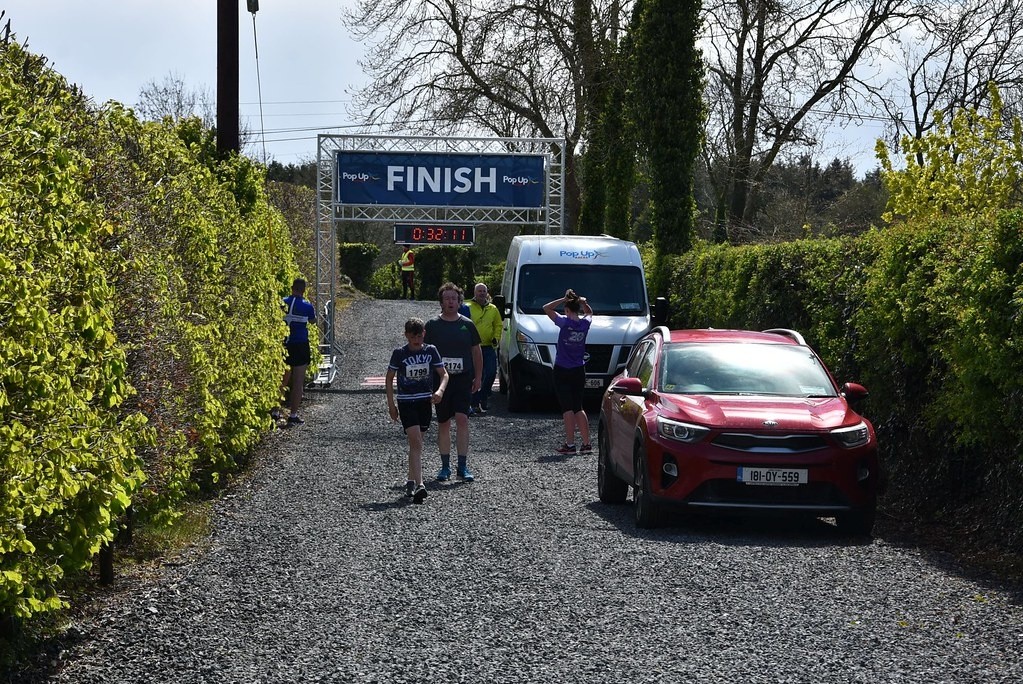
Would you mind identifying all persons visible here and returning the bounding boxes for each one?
[423,283,482,481]
[456,287,470,318]
[386,319,450,503]
[269,278,317,423]
[542,294,594,454]
[465,283,503,413]
[398,244,415,300]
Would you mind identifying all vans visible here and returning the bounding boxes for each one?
[492,233,668,415]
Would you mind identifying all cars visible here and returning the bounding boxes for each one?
[596,324,884,542]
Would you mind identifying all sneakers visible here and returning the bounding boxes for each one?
[555,442,576,454]
[437,470,451,481]
[456,469,473,482]
[577,442,593,454]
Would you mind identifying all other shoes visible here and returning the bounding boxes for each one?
[405,481,414,496]
[287,416,304,424]
[410,297,416,301]
[272,414,280,421]
[399,298,407,301]
[413,489,428,505]
[478,396,489,412]
